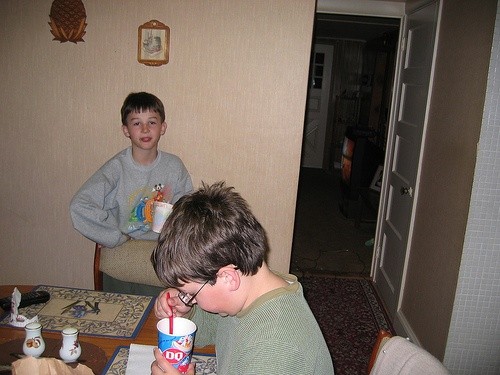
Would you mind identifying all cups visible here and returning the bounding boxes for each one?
[151,202,174,233]
[157,317,198,374]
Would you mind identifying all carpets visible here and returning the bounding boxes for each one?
[298,277,397,375]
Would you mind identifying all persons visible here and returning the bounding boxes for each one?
[69,90,194,296]
[149,181,334,375]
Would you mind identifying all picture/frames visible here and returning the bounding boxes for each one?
[137,19,171,66]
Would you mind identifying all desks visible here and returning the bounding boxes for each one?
[0,283,216,375]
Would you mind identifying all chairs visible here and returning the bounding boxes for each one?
[93,240,165,297]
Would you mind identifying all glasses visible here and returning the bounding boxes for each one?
[178,266,239,307]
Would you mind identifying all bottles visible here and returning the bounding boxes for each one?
[23,323,45,358]
[59,327,82,362]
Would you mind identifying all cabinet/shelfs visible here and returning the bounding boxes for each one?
[329,95,360,173]
